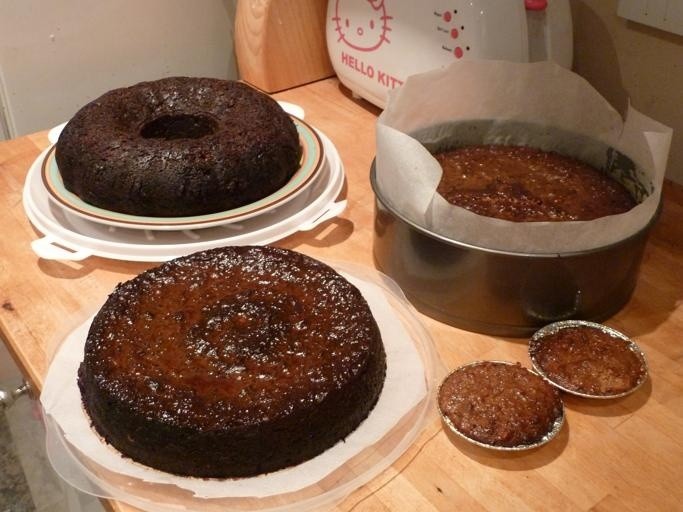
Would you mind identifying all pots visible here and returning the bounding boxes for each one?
[370,117,664,342]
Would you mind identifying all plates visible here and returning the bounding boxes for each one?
[42,108,327,234]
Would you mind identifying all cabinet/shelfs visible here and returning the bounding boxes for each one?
[0,78,683,512]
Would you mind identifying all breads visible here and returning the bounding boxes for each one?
[426,145,638,238]
[54,76,300,215]
[532,326,646,395]
[77,244,386,481]
[440,362,562,447]
